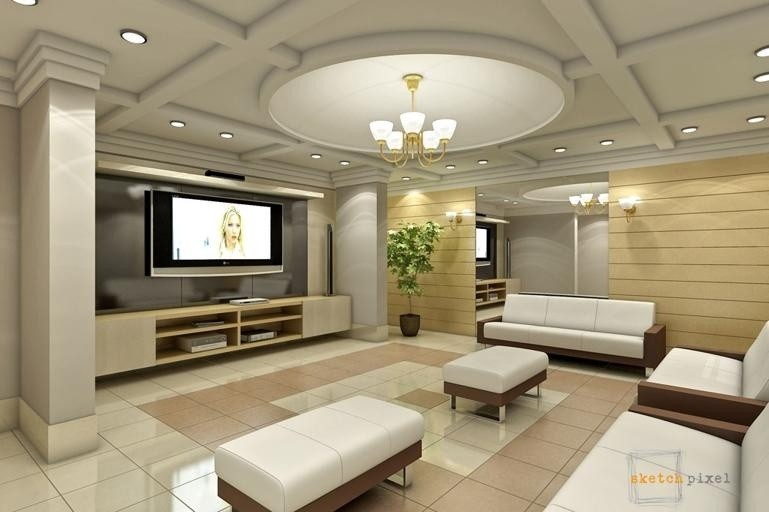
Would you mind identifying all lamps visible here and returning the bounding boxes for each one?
[367,73,457,167]
[569,188,608,216]
[617,196,637,222]
[445,211,461,230]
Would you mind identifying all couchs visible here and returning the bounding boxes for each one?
[477,294,666,377]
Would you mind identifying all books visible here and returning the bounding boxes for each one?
[192,319,225,326]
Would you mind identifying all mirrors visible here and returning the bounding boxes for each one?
[474,171,609,337]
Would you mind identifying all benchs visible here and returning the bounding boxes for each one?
[213,395,425,512]
[443,346,550,424]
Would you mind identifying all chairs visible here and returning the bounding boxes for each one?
[543,322,769,512]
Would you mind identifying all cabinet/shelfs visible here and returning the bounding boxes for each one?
[476,278,520,305]
[95,292,352,377]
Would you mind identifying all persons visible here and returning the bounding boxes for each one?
[209,206,246,259]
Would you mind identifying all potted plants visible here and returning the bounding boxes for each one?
[387,220,444,338]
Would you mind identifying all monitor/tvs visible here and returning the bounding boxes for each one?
[476,221,497,279]
[150,189,283,278]
